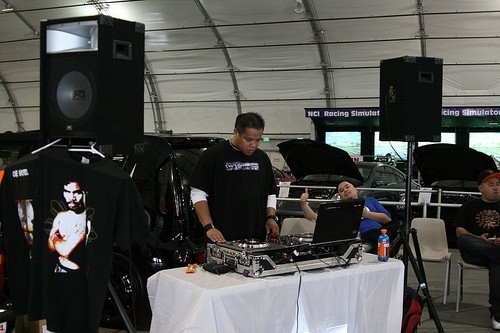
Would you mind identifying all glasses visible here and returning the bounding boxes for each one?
[243,136,263,144]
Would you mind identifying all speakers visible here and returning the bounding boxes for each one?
[379,55,443,143]
[39,14,144,144]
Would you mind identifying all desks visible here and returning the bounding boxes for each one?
[147,252,405,333]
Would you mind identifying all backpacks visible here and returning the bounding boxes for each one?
[401,293,425,333]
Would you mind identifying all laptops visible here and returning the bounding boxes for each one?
[295,198,366,243]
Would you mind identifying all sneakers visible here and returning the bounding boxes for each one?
[491,317,500,330]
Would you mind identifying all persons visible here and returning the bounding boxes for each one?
[456,169,500,329]
[47,176,91,273]
[189,113,280,262]
[300,180,392,254]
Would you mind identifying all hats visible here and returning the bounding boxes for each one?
[477,169,500,185]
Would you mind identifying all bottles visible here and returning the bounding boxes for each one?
[378,229,389,261]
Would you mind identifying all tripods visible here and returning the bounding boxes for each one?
[389,142,444,333]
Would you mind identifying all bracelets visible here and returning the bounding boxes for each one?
[203,222,214,233]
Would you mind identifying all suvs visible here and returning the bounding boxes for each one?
[0,128,227,330]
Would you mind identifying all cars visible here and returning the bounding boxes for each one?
[271,138,500,247]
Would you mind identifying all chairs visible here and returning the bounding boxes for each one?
[280,218,316,236]
[395,218,500,312]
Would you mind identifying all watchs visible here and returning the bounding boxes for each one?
[267,215,279,223]
